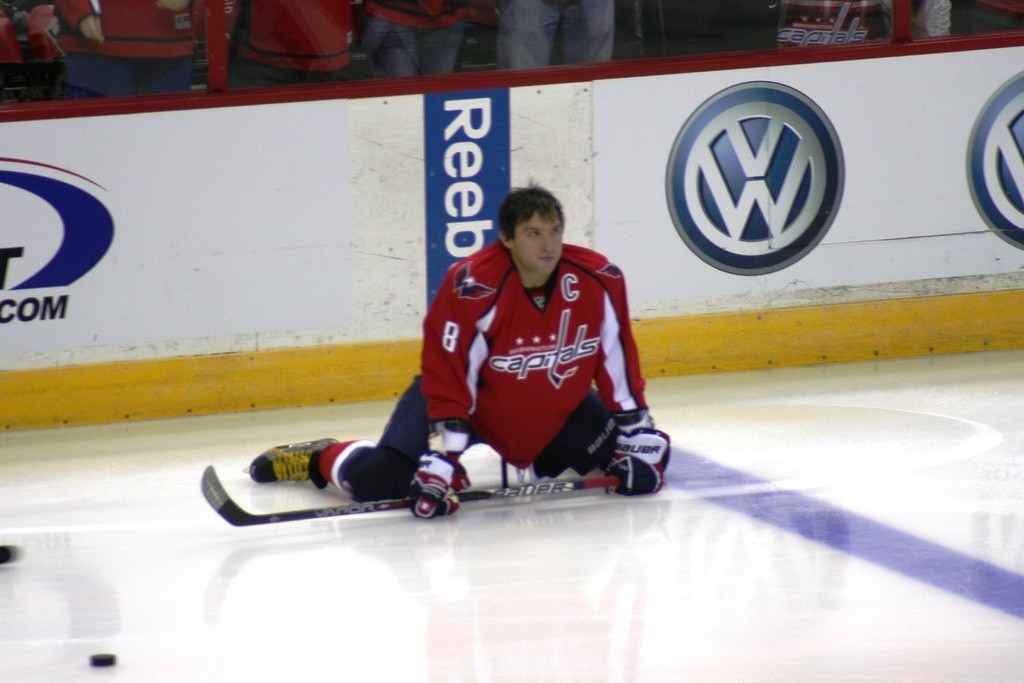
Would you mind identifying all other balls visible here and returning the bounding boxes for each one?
[89,653,116,667]
[0,545,11,564]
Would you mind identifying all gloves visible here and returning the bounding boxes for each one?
[604,406,671,496]
[408,419,471,519]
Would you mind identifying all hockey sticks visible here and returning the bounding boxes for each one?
[199,465,621,529]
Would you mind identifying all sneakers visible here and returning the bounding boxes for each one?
[242,439,341,489]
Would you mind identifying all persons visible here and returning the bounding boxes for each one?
[877,0,952,40]
[250,185,671,519]
[491,0,618,70]
[0,0,474,100]
